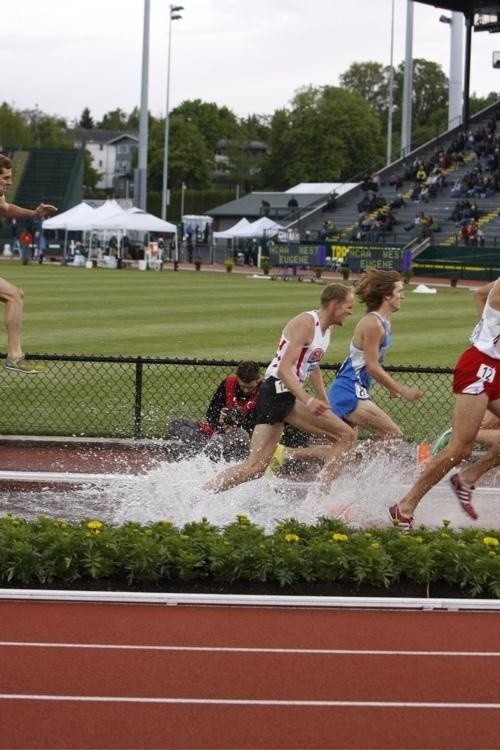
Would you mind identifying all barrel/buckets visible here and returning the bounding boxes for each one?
[138,260,148,271]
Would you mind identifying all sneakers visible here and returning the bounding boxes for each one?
[387,503,414,537]
[4,355,49,374]
[269,443,284,480]
[447,473,478,521]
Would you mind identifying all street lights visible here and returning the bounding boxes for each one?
[161,0,187,222]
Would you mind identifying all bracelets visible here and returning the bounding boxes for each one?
[305,396,313,407]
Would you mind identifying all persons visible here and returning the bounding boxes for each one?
[326,260,421,485]
[191,283,358,494]
[146,222,212,262]
[9,217,17,250]
[205,361,337,482]
[431,406,500,457]
[20,227,33,265]
[0,154,58,374]
[387,274,500,536]
[73,233,132,261]
[35,232,46,264]
